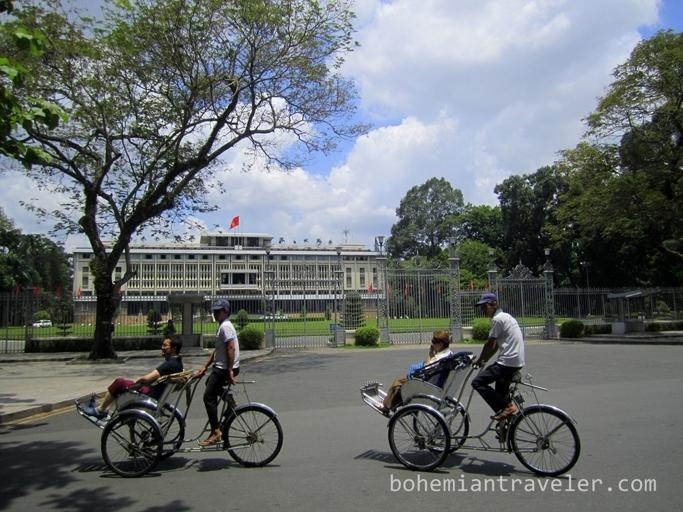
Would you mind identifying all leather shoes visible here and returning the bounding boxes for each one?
[490,401,516,419]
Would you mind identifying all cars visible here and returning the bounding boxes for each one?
[33,319,53,328]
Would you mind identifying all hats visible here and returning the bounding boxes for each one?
[212,300,230,311]
[475,293,496,306]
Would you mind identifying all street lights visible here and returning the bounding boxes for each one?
[334,246,345,331]
[487,247,499,296]
[265,244,274,331]
[376,235,386,327]
[542,247,556,338]
[448,236,462,328]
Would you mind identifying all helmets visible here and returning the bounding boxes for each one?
[79,402,108,420]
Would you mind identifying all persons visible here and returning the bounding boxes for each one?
[191,298,241,446]
[471,292,526,444]
[77,334,184,418]
[374,329,454,417]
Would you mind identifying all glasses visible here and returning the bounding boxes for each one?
[432,340,442,344]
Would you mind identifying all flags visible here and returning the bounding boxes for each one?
[366,280,491,300]
[16,286,82,303]
[228,216,239,229]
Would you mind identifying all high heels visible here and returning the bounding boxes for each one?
[197,432,222,446]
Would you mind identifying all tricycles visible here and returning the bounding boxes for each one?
[75,369,284,477]
[360,352,582,477]
